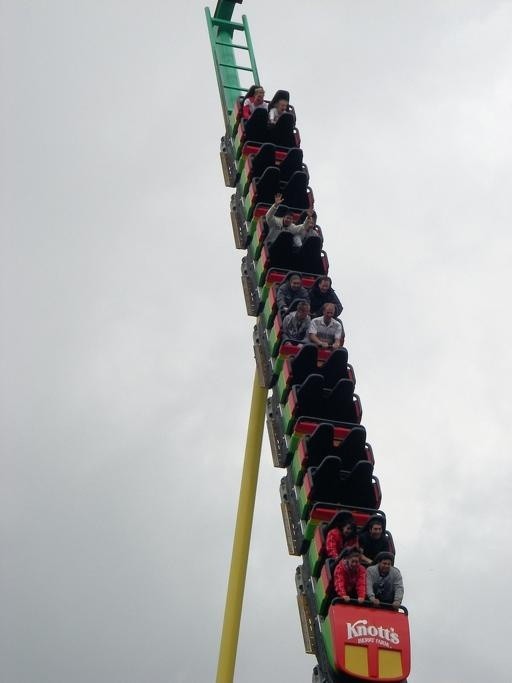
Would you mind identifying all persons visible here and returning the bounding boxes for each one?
[324,518,358,559]
[309,302,342,367]
[269,98,289,123]
[308,274,343,317]
[358,519,393,560]
[364,553,404,608]
[276,273,310,316]
[293,216,319,245]
[333,552,368,604]
[265,192,313,245]
[241,87,268,120]
[280,301,312,346]
